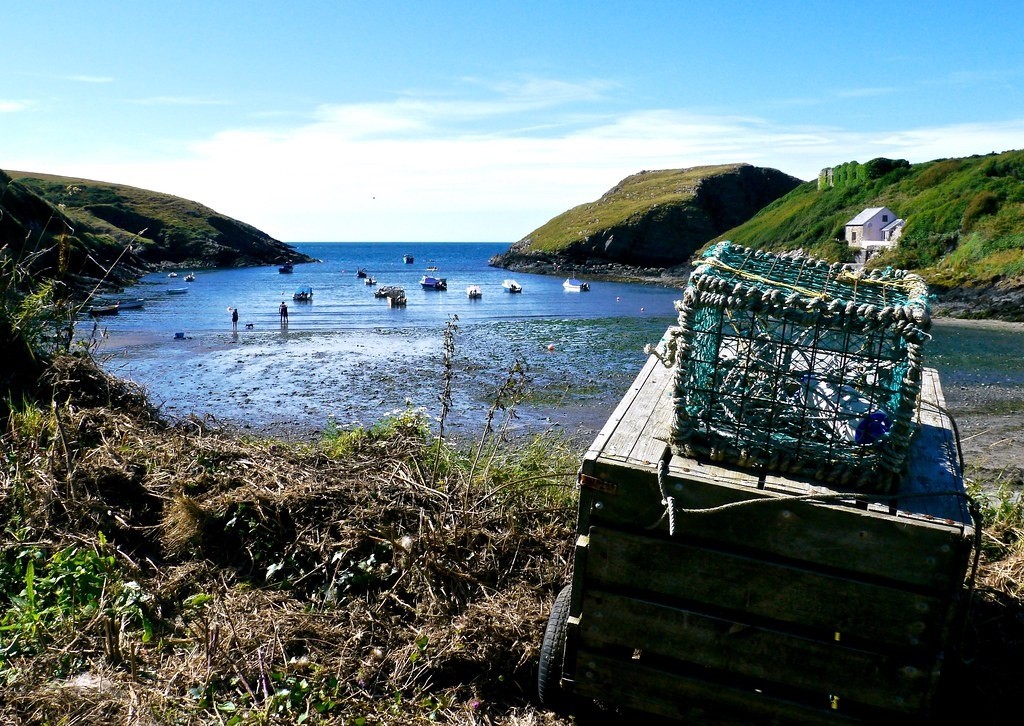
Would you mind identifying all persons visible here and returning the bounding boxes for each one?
[279,302,288,322]
[231,309,239,327]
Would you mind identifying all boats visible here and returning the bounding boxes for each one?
[105,298,145,308]
[419,275,437,288]
[426,265,438,272]
[403,255,415,264]
[433,278,447,290]
[167,286,189,295]
[375,284,408,307]
[185,273,196,281]
[465,286,482,298]
[294,286,313,301]
[279,261,293,273]
[168,271,177,277]
[86,303,120,316]
[563,277,589,292]
[354,267,367,277]
[502,279,522,292]
[364,276,378,285]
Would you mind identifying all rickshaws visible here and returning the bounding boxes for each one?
[535,328,984,726]
